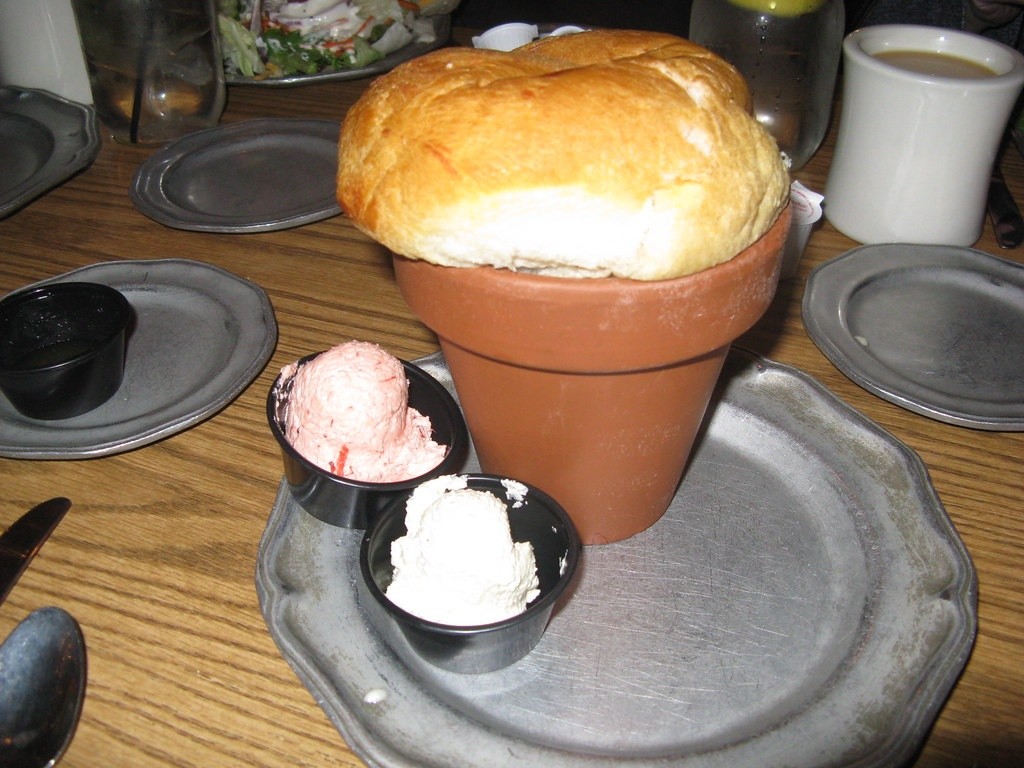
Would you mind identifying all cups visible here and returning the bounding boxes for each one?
[689,0,845,175]
[825,25,1024,248]
[782,222,813,280]
[70,0,226,145]
[0,0,96,106]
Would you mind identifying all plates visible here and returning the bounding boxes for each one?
[0,85,99,218]
[82,11,453,85]
[257,347,980,768]
[130,118,345,232]
[0,258,282,460]
[802,243,1024,432]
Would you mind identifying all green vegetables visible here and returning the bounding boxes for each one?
[218,15,382,74]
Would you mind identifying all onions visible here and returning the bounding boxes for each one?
[250,0,435,54]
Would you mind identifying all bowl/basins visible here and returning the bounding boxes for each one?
[266,348,465,529]
[0,281,132,422]
[360,475,581,673]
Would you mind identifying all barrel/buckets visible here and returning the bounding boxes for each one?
[392,192,793,543]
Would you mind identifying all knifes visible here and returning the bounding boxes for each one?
[987,161,1024,248]
[0,485,71,604]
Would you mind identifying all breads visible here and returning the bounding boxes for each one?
[336,28,791,281]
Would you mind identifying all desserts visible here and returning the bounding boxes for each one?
[281,341,541,626]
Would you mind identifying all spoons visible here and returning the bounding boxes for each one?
[0,607,86,768]
